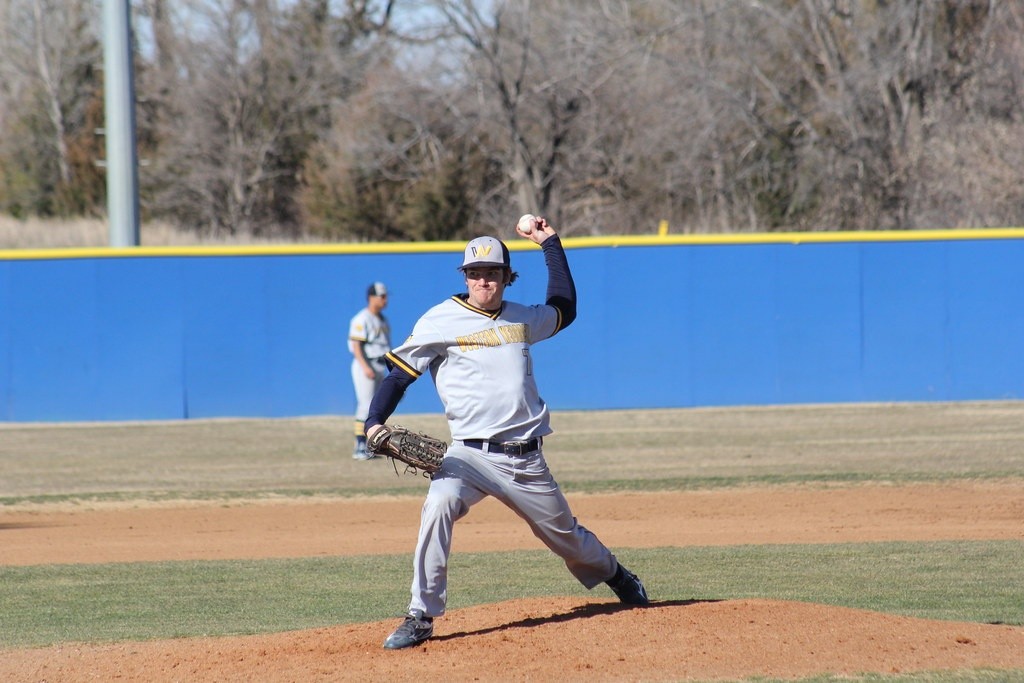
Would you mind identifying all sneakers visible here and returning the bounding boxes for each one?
[603,563,649,606]
[383,609,434,649]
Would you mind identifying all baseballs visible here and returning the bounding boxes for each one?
[519,214,538,234]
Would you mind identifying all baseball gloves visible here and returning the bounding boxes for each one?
[366,424,448,473]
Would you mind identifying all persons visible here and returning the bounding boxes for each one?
[348,281,392,460]
[364,215,647,649]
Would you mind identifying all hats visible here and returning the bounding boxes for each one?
[368,282,388,296]
[462,236,510,267]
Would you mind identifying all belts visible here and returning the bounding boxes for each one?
[463,436,543,458]
[369,357,386,364]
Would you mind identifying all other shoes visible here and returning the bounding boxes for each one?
[352,449,375,460]
